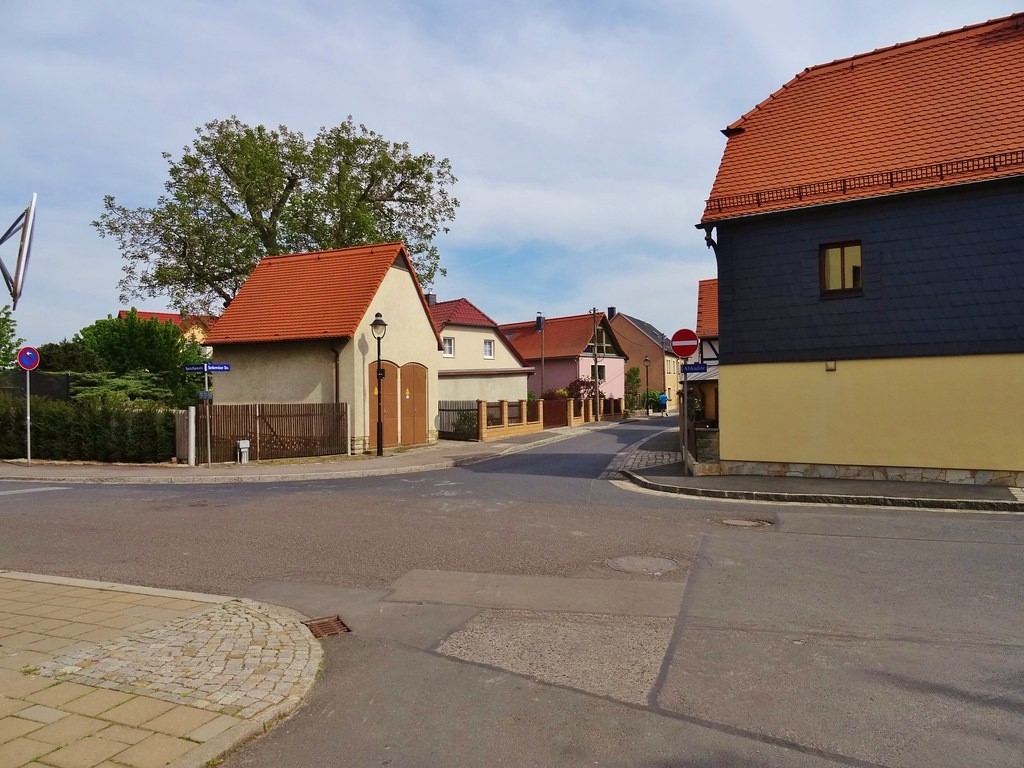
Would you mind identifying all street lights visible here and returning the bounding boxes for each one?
[643,355,651,416]
[370,312,389,456]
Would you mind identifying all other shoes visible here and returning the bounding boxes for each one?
[666,413,668,416]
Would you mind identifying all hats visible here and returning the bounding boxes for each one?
[662,391,664,393]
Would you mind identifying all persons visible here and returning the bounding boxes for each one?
[659,392,672,417]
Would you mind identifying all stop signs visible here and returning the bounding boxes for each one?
[671,329,700,358]
